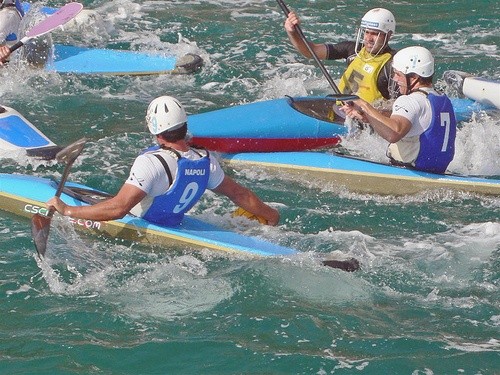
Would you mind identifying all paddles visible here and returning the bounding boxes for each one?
[324,93,364,117]
[8,2,84,54]
[277,0,366,131]
[29,136,89,262]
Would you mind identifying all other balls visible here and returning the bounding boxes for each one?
[231,206,267,230]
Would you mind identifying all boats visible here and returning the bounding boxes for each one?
[0,41,204,75]
[15,0,96,16]
[140,144,500,198]
[0,103,63,161]
[1,173,361,272]
[186,95,500,152]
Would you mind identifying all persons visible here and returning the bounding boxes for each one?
[46,95,281,225]
[0,0,26,68]
[284,8,455,173]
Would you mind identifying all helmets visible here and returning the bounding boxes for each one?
[360,8,396,34]
[392,45,435,78]
[146,96,188,135]
